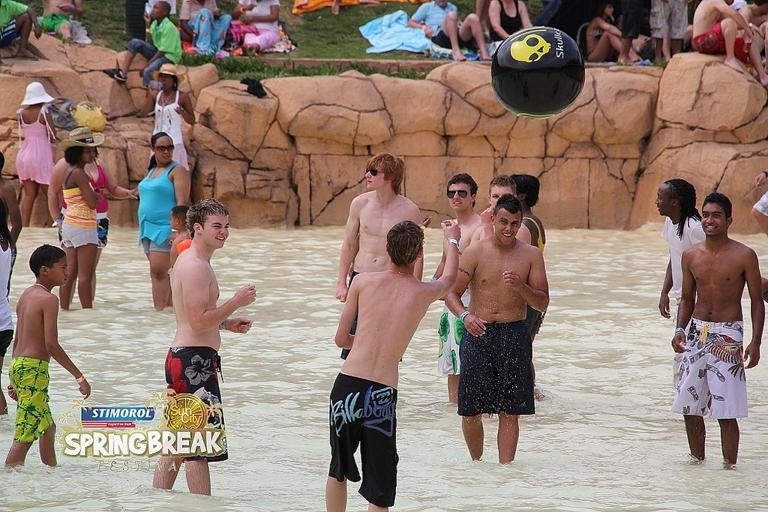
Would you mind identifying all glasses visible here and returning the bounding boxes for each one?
[446,190,468,198]
[366,167,383,176]
[152,144,173,152]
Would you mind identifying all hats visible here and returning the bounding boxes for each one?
[21,82,55,105]
[58,127,106,146]
[153,64,186,85]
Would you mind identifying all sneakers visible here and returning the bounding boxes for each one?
[104,68,127,83]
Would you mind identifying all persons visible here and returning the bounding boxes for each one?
[1,0,41,61]
[0,197,14,415]
[179,0,232,58]
[692,0,768,86]
[47,157,138,303]
[671,194,764,466]
[32,0,84,41]
[153,199,256,495]
[738,2,768,75]
[15,83,57,228]
[337,154,424,362]
[432,173,481,404]
[650,0,692,62]
[4,244,90,466]
[761,278,768,302]
[475,1,490,38]
[168,206,191,275]
[471,174,531,244]
[655,179,706,318]
[618,0,644,65]
[444,194,549,464]
[118,0,149,42]
[486,0,533,58]
[141,64,195,171]
[326,219,461,512]
[408,0,492,61]
[509,174,546,401]
[110,1,183,116]
[294,0,343,16]
[59,127,99,311]
[138,132,190,312]
[751,168,768,237]
[0,151,23,303]
[585,4,645,62]
[232,0,280,56]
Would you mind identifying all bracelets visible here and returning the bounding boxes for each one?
[762,171,768,177]
[77,374,85,384]
[448,239,459,250]
[221,320,225,329]
[675,328,685,333]
[459,311,469,323]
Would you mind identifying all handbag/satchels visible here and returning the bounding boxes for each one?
[74,102,106,132]
